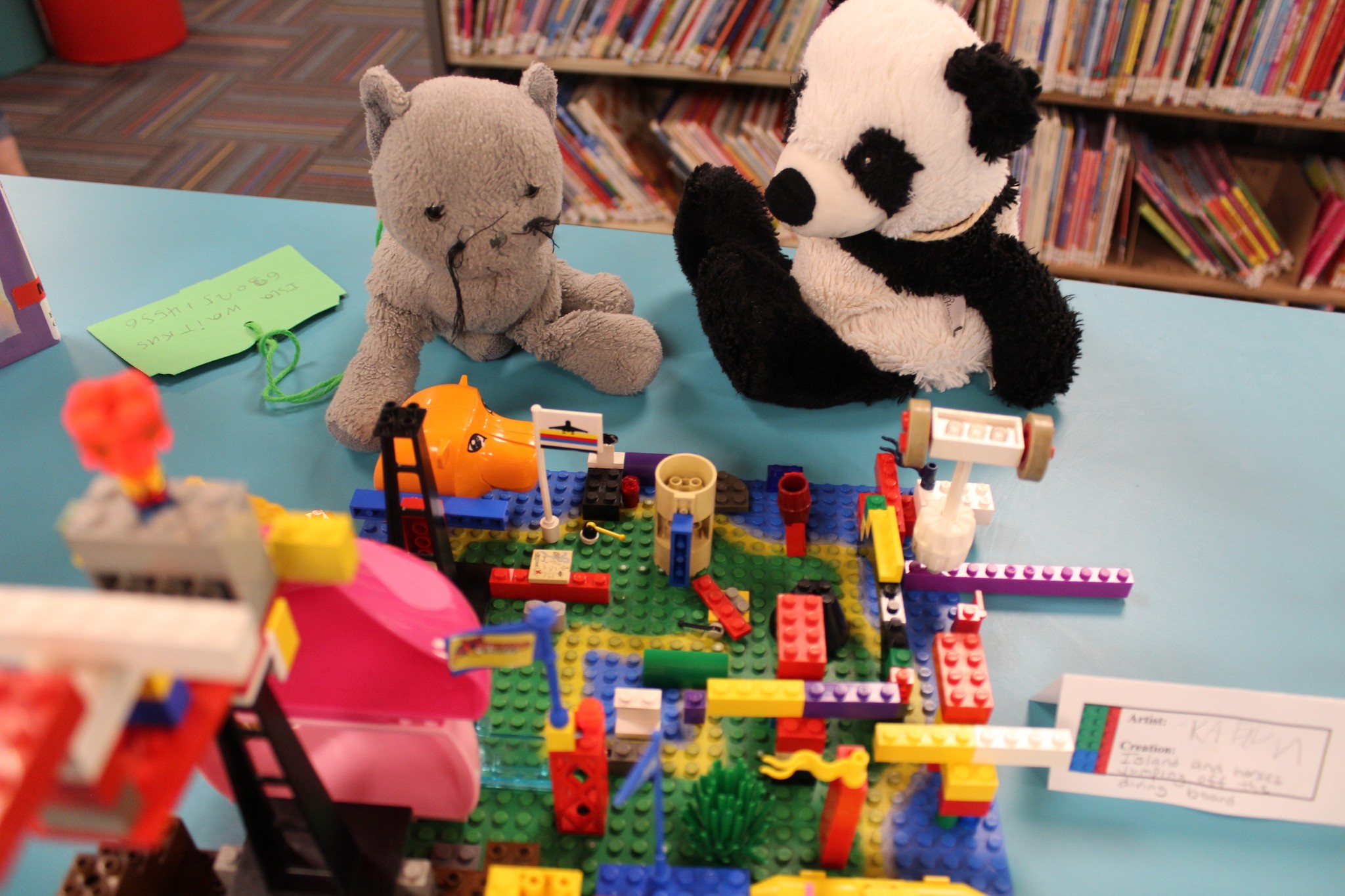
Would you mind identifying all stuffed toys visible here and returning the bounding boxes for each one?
[322,62,663,453]
[671,0,1084,410]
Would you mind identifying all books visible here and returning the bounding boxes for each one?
[436,1,1345,291]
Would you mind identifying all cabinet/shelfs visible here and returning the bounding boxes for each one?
[432,0,1345,313]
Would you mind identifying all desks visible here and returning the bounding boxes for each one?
[0,175,1345,896]
